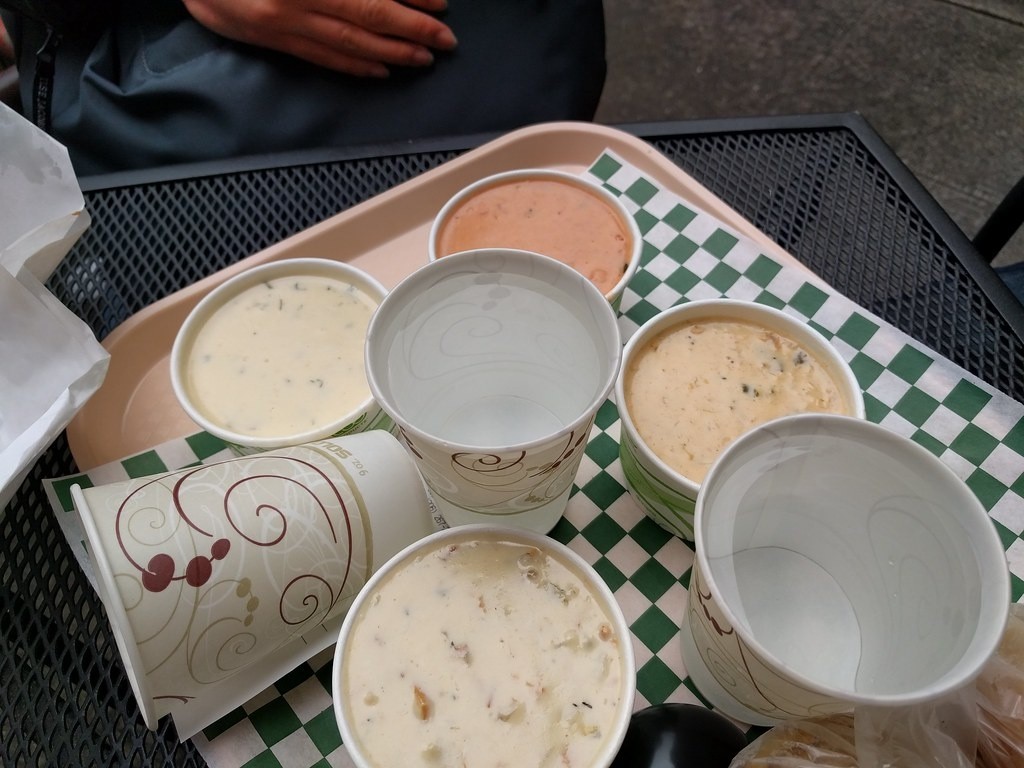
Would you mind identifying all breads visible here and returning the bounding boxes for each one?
[732,619,1024,768]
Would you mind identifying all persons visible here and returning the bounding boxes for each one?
[182,0,456,77]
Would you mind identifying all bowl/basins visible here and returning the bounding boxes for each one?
[615,298,864,542]
[333,523,636,767]
[428,168,642,316]
[170,258,391,459]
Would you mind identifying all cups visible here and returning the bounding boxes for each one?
[69,430,433,732]
[681,412,1012,726]
[363,248,622,534]
